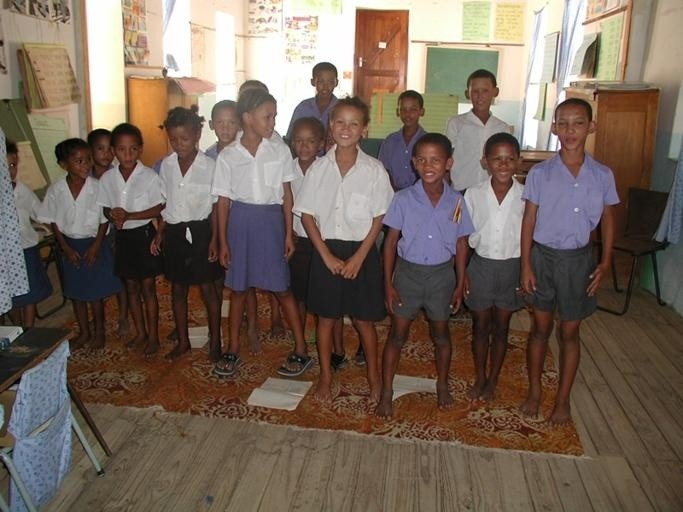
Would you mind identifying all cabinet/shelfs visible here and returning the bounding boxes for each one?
[128,76,199,172]
[562,83,660,291]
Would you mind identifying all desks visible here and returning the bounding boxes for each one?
[0,325,114,512]
[515,149,558,186]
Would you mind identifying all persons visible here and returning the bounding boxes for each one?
[379,90,431,270]
[462,131,528,401]
[519,97,622,423]
[38,137,110,349]
[447,68,511,317]
[374,131,475,422]
[88,129,132,340]
[4,138,54,327]
[98,122,168,359]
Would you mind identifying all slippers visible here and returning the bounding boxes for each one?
[214,352,241,376]
[276,354,315,376]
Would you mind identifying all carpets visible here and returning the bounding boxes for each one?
[20,272,591,461]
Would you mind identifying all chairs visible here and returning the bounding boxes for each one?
[0,339,106,512]
[591,186,669,315]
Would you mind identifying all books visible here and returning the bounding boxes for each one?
[17,42,81,110]
[0,325,24,352]
